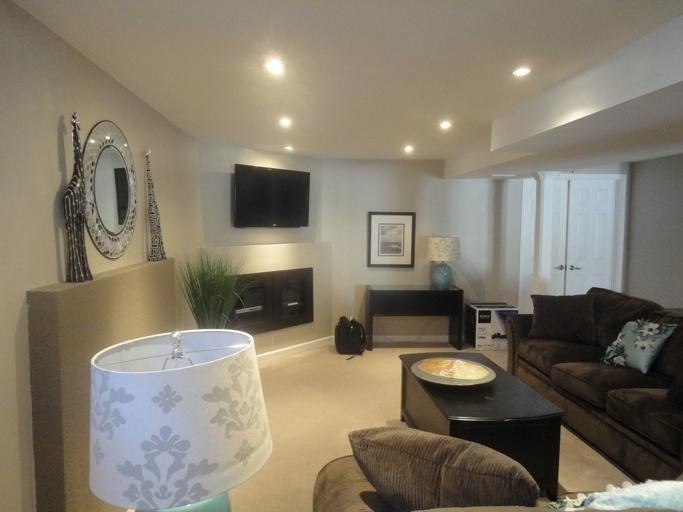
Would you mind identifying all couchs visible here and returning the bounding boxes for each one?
[309,425,682,512]
[504,285,682,487]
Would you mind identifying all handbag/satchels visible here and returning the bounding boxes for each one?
[335,315,367,355]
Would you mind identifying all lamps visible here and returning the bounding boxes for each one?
[426,236,461,288]
[87,329,274,511]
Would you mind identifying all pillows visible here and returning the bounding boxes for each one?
[529,293,596,344]
[601,318,677,376]
[346,425,540,511]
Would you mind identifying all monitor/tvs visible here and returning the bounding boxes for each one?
[114,168,128,224]
[235,164,309,227]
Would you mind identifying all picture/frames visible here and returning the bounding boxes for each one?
[366,211,416,267]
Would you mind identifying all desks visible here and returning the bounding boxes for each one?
[365,285,464,350]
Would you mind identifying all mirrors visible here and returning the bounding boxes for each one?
[81,119,138,261]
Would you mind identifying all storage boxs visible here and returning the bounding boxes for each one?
[466,302,518,351]
